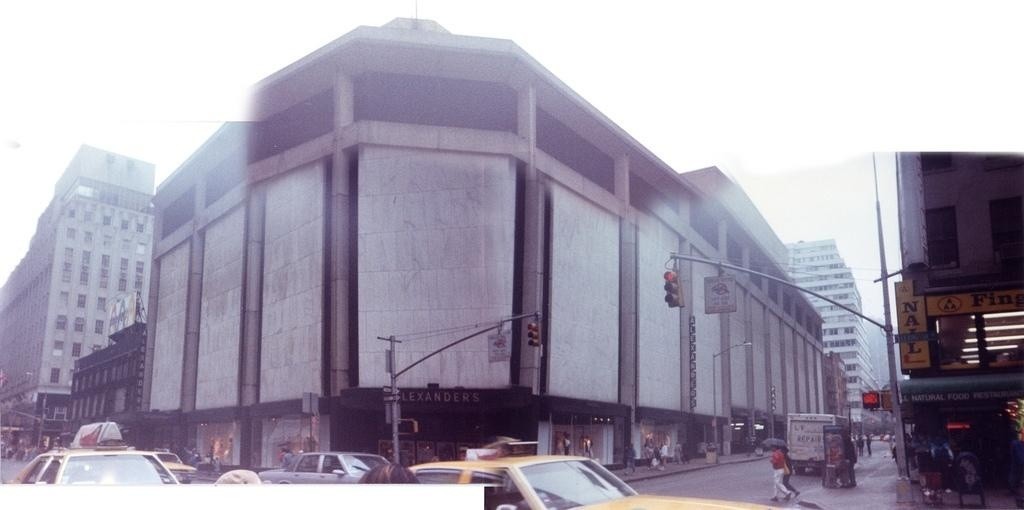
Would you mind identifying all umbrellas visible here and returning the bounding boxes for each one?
[760,437,787,447]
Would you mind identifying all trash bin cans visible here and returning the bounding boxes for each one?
[920,471,943,505]
[706,446,717,464]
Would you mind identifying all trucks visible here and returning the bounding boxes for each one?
[786,412,858,477]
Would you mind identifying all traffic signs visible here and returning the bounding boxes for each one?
[383,394,401,403]
[382,386,399,394]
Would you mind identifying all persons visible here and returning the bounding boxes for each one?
[555,431,564,455]
[888,428,1023,498]
[279,447,286,467]
[354,462,420,484]
[562,431,571,455]
[823,428,874,490]
[583,436,593,458]
[780,445,801,499]
[768,444,790,502]
[153,444,221,476]
[621,439,686,475]
[281,449,291,468]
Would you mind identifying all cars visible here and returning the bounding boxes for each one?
[257,450,393,486]
[8,448,183,487]
[138,450,198,485]
[407,454,780,509]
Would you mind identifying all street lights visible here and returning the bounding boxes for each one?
[23,369,49,452]
[712,339,755,464]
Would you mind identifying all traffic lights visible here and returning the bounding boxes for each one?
[527,320,541,350]
[664,270,685,309]
[861,390,880,410]
